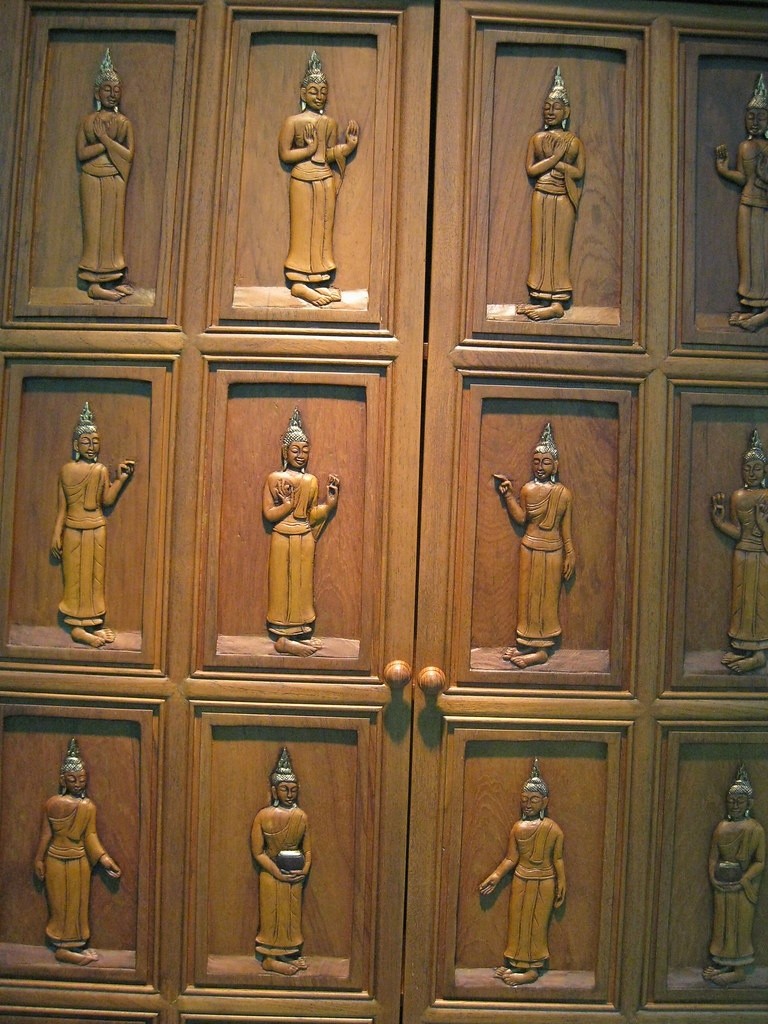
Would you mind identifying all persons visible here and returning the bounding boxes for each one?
[710,427,768,674]
[715,74,768,333]
[74,48,136,300]
[516,65,587,320]
[251,748,312,975]
[491,422,576,667]
[263,408,341,657]
[478,759,567,986]
[702,763,766,986]
[49,401,135,647]
[35,737,122,967]
[279,51,359,306]
